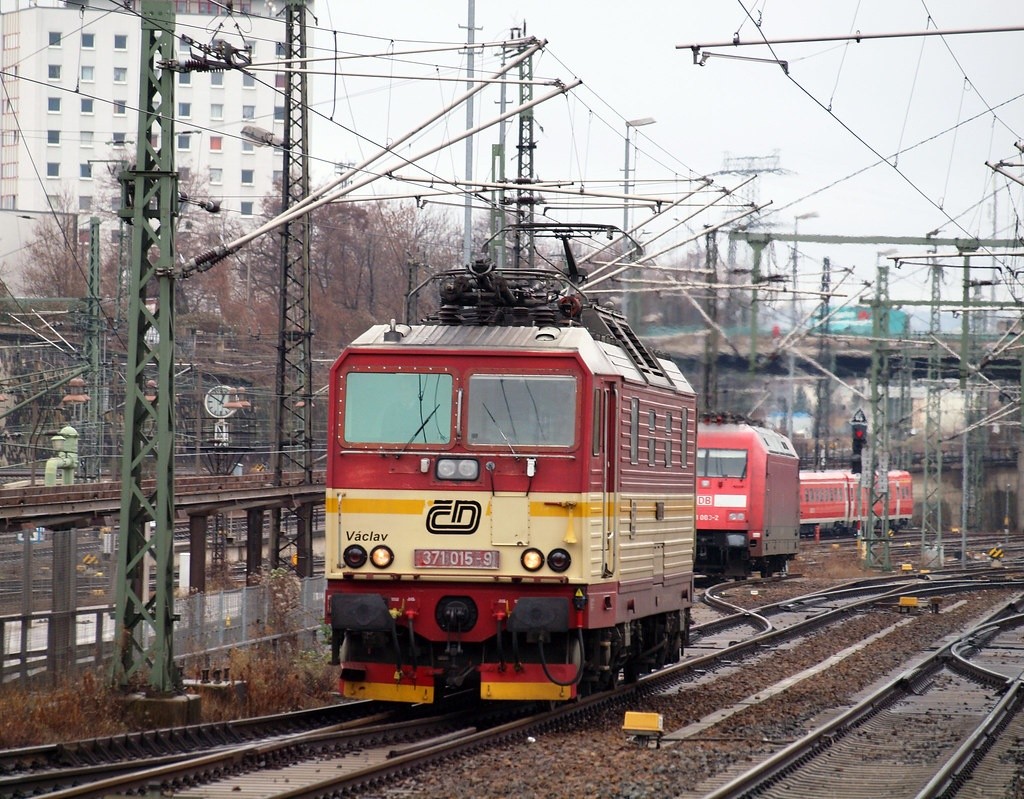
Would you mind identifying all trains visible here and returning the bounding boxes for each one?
[326,262,698,714]
[692,410,912,578]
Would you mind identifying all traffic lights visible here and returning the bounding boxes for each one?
[848,421,867,474]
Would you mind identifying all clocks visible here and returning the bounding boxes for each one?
[203,385,241,419]
